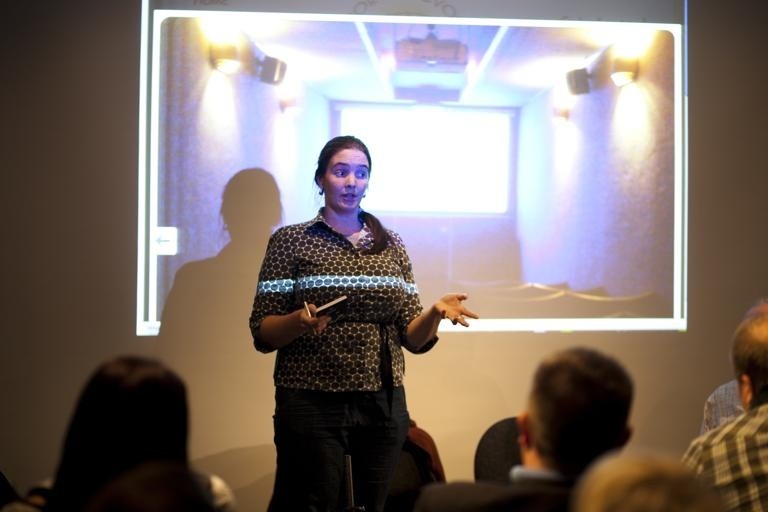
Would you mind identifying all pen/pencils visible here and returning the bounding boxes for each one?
[303,301,317,336]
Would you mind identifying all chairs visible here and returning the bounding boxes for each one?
[391,414,447,510]
[472,416,522,484]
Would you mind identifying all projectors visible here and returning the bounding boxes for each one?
[396,38,459,60]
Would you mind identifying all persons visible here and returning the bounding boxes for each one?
[1,355,237,512]
[475,303,768,512]
[246,134,483,512]
